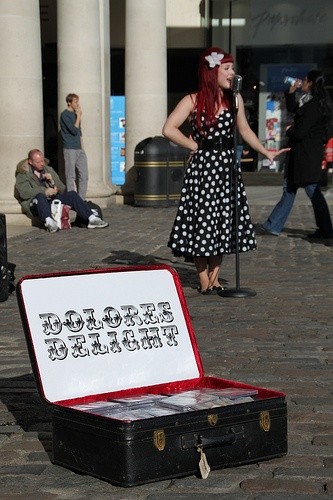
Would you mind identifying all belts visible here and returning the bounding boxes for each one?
[198,136,234,152]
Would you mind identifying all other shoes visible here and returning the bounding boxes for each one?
[214,286,224,291]
[308,229,332,238]
[199,285,213,295]
[256,223,280,236]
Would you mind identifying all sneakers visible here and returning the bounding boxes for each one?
[44,220,59,233]
[87,217,109,228]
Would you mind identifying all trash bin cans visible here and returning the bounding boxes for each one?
[134,135,187,207]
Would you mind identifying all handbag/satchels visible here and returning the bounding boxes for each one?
[50,199,71,230]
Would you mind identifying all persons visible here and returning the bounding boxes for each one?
[258,69,333,246]
[164,46,291,293]
[14,149,109,229]
[61,93,88,201]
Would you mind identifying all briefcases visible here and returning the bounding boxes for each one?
[16,264,288,488]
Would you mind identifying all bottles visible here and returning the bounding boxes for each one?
[284,76,303,88]
